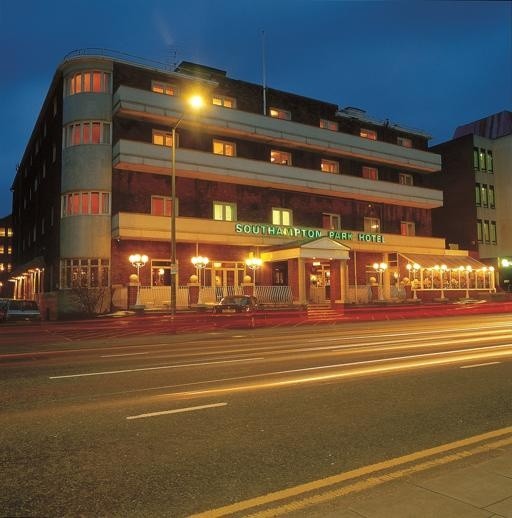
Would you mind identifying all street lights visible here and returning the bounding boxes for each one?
[433,264,449,299]
[373,262,388,302]
[128,254,150,307]
[481,265,495,294]
[458,264,474,299]
[406,263,421,300]
[166,92,208,325]
[191,255,210,303]
[244,256,265,296]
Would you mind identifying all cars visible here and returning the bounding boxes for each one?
[0,298,12,321]
[5,298,43,323]
[210,294,269,329]
